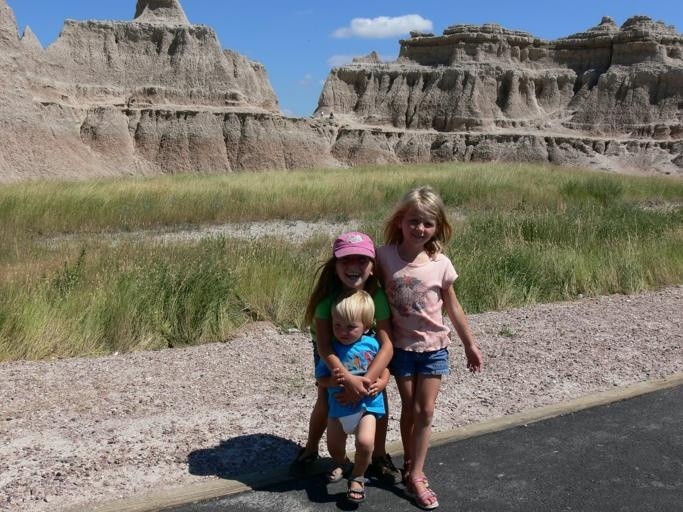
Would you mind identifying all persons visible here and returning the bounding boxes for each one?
[367,185,483,510]
[310,289,389,502]
[285,231,402,485]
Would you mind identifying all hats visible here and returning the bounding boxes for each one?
[331,232,376,261]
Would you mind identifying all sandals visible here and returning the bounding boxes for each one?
[327,458,352,484]
[348,477,366,503]
[404,456,441,509]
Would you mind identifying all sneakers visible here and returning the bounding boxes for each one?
[289,446,317,480]
[372,452,403,485]
